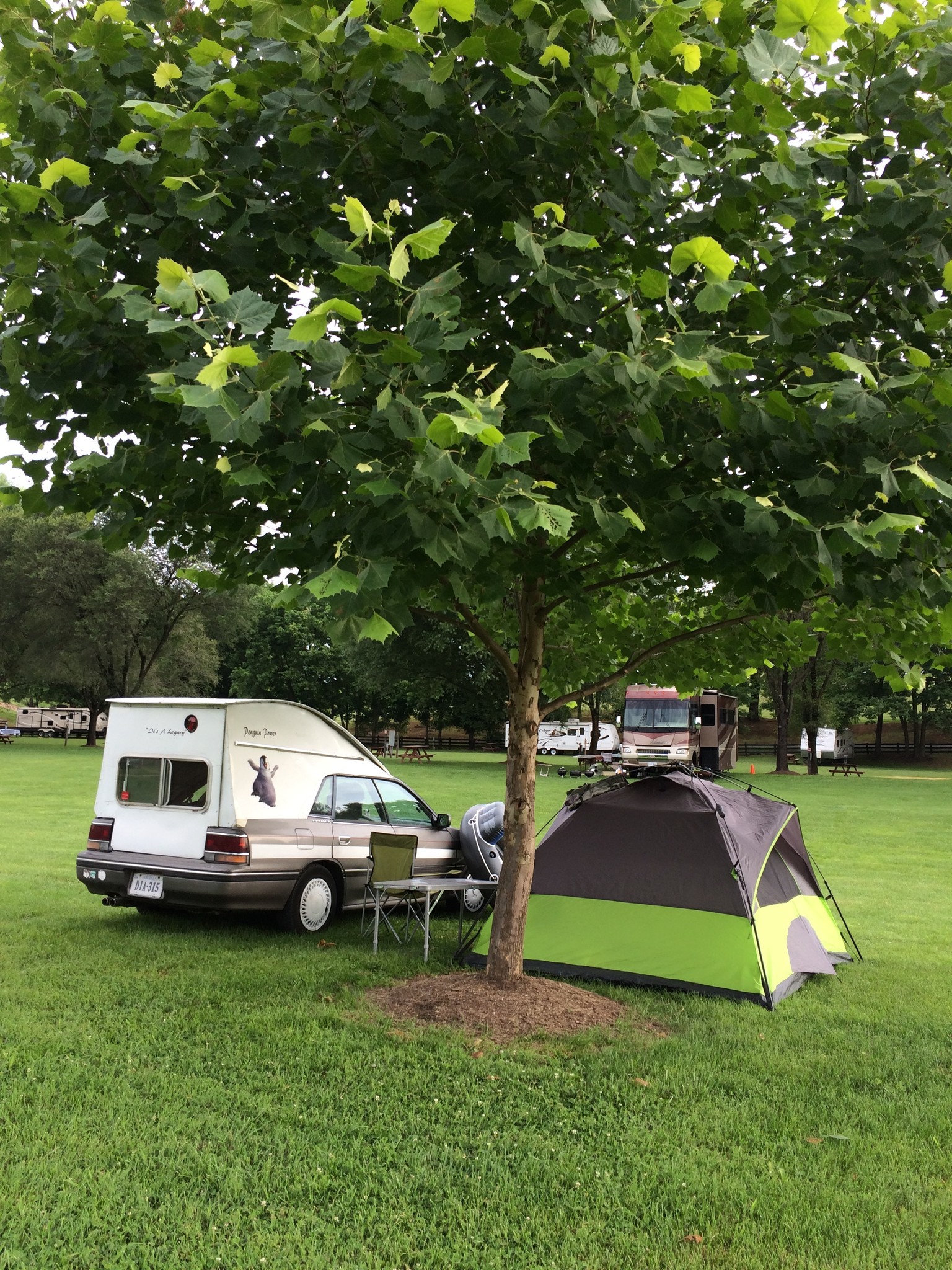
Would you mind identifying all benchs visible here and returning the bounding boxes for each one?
[398,754,436,759]
[372,753,395,756]
[0,740,14,742]
[794,758,800,761]
[481,747,499,750]
[827,769,863,774]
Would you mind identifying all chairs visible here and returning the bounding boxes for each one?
[360,832,462,944]
[601,752,612,771]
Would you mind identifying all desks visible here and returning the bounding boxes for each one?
[573,755,603,771]
[537,764,552,777]
[0,735,12,745]
[612,754,621,762]
[0,729,21,738]
[371,747,390,758]
[373,878,498,963]
[482,743,496,753]
[787,754,798,764]
[832,763,860,777]
[400,745,431,764]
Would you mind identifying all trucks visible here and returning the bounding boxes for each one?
[15,707,108,738]
[505,718,620,755]
[615,684,738,776]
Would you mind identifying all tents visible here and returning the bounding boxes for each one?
[454,760,864,1012]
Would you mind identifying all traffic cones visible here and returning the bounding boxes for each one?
[750,764,755,775]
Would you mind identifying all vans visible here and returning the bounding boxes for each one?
[75,695,487,934]
